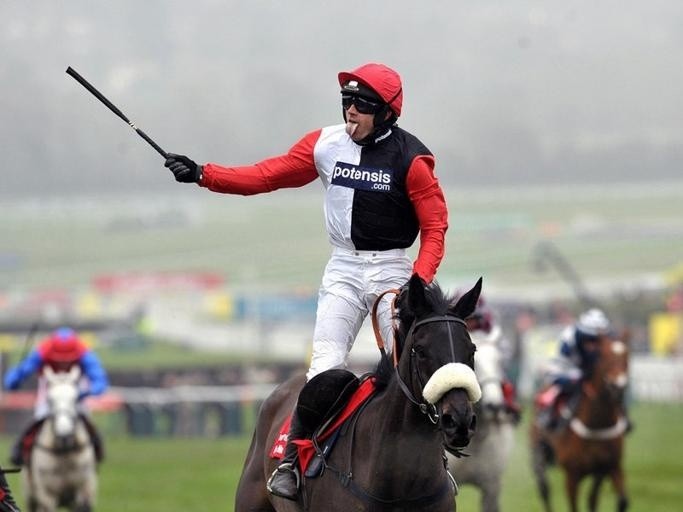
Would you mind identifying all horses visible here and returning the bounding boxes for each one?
[19,365,98,512]
[528,326,629,512]
[234,272,483,512]
[443,325,514,512]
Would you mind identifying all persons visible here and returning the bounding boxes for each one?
[0,328,110,473]
[100,357,305,438]
[163,65,448,498]
[452,290,522,426]
[528,308,634,437]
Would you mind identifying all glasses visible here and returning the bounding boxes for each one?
[342,93,377,115]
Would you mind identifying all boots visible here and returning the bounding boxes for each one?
[271,405,303,499]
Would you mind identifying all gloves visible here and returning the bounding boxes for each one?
[394,277,426,310]
[164,152,203,182]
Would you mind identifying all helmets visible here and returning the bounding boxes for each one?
[577,308,610,336]
[51,327,79,362]
[338,63,403,116]
